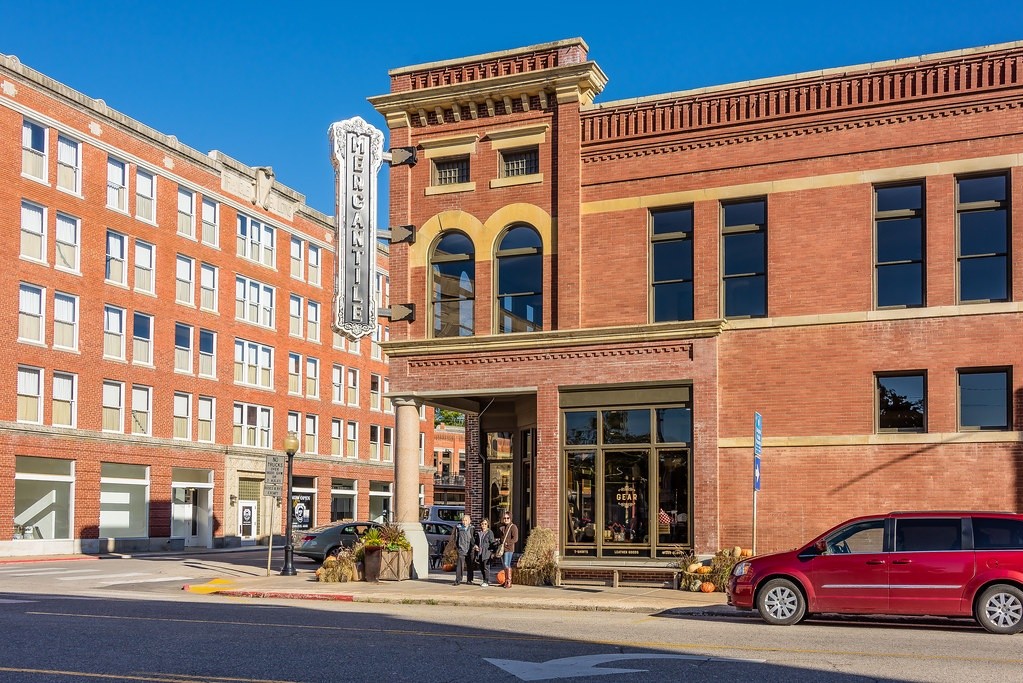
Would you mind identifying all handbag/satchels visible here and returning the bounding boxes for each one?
[495,544,504,557]
[473,551,480,567]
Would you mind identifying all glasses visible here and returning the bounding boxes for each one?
[503,517,510,519]
[481,523,487,525]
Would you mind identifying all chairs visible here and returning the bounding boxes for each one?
[429,540,449,570]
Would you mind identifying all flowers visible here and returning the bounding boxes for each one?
[351,521,412,556]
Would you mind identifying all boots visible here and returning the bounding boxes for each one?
[504,567,512,587]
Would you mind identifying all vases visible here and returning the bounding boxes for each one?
[363,547,413,581]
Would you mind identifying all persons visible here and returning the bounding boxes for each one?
[453,514,476,585]
[498,512,520,589]
[475,516,495,587]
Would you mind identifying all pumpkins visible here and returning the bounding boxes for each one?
[442,563,453,572]
[496,570,505,584]
[715,545,752,557]
[689,580,715,593]
[315,555,337,576]
[687,563,713,573]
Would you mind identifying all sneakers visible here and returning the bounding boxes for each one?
[480,582,488,586]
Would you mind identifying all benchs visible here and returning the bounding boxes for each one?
[557,565,684,590]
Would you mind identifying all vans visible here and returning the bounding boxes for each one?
[725,510,1023,635]
[426,504,466,534]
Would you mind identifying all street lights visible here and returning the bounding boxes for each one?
[279,430,299,576]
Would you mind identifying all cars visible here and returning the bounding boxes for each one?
[293,521,385,564]
[420,520,455,559]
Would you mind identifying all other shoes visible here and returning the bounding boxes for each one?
[466,580,472,584]
[454,580,460,585]
